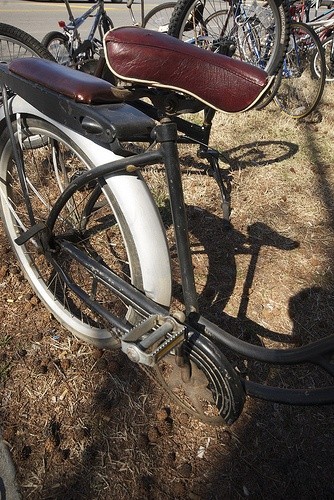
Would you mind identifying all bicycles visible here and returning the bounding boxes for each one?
[0,26,333,425]
[0,0,334,230]
